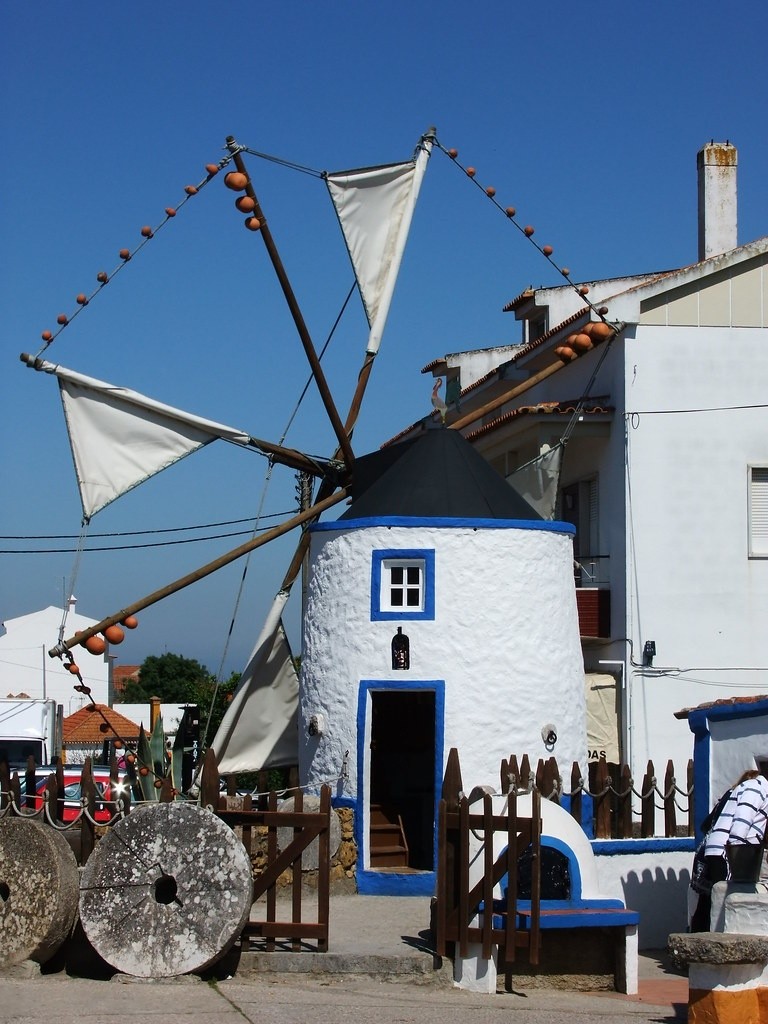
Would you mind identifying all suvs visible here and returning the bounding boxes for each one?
[5,763,135,829]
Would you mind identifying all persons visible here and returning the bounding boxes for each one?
[688,770,764,935]
[702,761,768,885]
